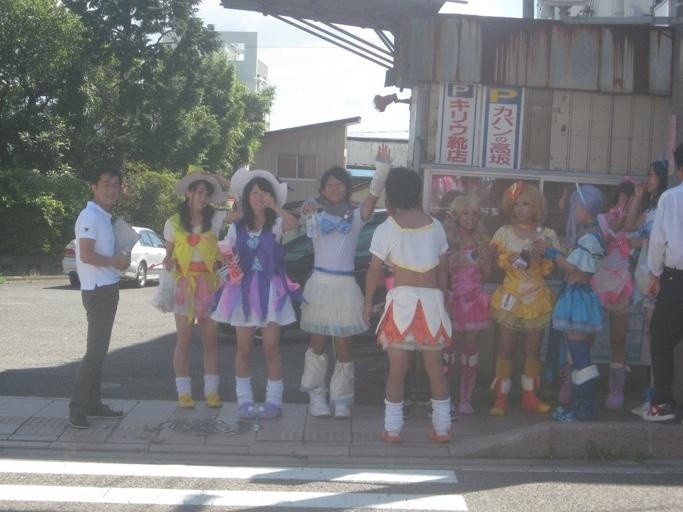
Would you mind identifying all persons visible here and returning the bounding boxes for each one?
[162,173,244,409]
[490,182,560,417]
[363,168,453,444]
[209,168,298,420]
[534,185,607,423]
[624,160,669,416]
[591,180,635,411]
[67,168,131,429]
[442,194,491,416]
[643,144,683,422]
[300,144,391,420]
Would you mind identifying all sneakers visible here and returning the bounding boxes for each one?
[88,404,125,419]
[403,399,415,419]
[642,402,676,422]
[69,415,90,429]
[425,400,459,422]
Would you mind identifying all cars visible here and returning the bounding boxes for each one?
[216,209,391,345]
[62,226,166,287]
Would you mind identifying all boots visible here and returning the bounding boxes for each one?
[521,374,551,412]
[300,348,331,419]
[458,352,479,414]
[442,352,456,413]
[331,361,355,419]
[631,386,656,417]
[203,374,223,409]
[236,377,257,418]
[427,397,451,443]
[258,378,284,418]
[606,363,631,410]
[490,378,511,415]
[551,405,568,420]
[383,397,404,442]
[175,378,196,408]
[561,364,599,423]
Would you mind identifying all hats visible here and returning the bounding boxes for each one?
[175,163,228,205]
[230,166,287,212]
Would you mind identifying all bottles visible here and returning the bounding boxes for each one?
[520,238,529,263]
[306,212,319,238]
[536,227,545,241]
